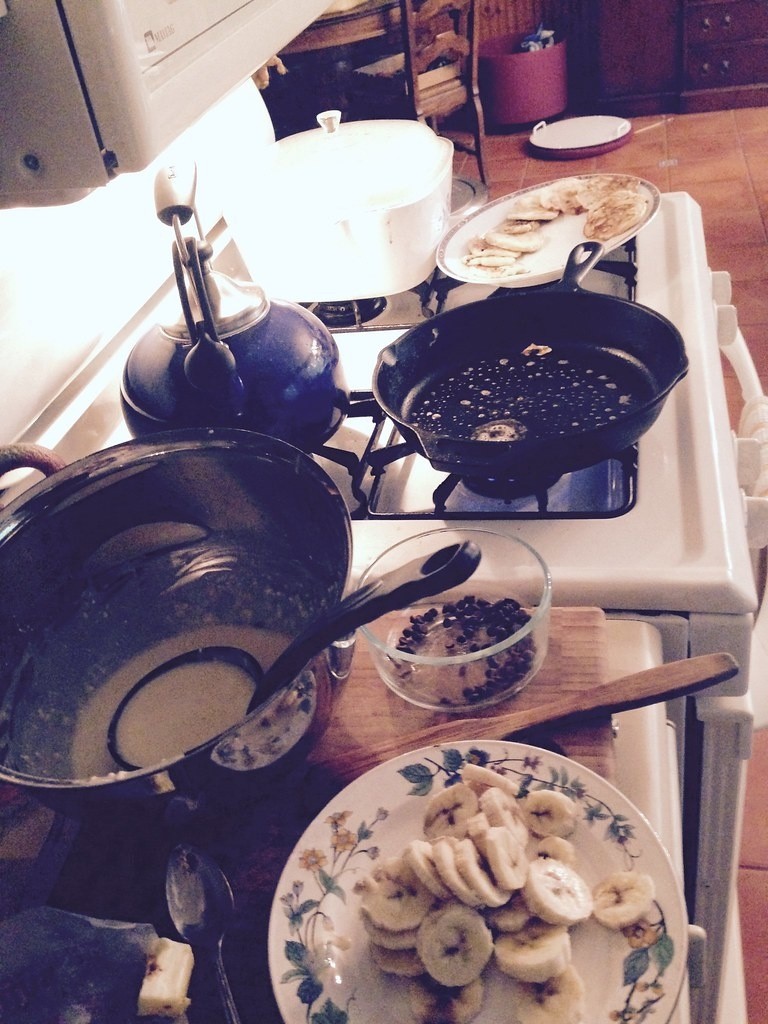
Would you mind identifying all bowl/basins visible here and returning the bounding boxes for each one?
[355,528,553,713]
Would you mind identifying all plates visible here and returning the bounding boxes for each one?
[267,740,688,1024]
[436,173,661,289]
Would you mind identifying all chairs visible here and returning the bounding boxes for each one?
[352,0,488,187]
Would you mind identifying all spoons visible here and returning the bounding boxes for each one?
[166,845,242,1024]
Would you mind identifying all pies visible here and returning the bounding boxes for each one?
[460,176,649,280]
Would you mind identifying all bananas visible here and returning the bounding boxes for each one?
[358,764,656,1024]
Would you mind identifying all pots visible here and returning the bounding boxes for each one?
[218,112,454,302]
[0,427,482,829]
[372,241,688,476]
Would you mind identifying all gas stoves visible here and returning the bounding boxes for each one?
[0,192,768,699]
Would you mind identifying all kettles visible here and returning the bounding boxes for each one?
[118,164,349,452]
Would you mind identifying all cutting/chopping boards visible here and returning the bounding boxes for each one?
[48,605,619,919]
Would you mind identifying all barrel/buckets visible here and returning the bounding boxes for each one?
[478,32,569,125]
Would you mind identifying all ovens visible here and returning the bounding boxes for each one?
[690,270,768,1024]
